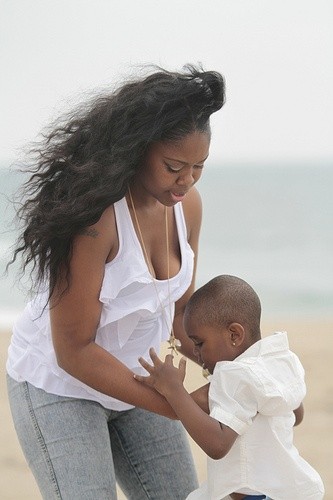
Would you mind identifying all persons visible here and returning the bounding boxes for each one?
[5,62,225,500]
[132,275,324,500]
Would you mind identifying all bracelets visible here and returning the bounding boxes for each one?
[202,362,210,379]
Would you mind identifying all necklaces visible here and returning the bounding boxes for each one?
[126,179,181,357]
